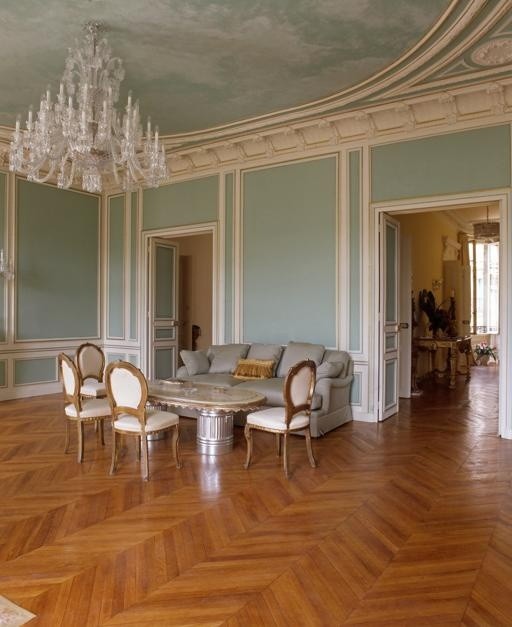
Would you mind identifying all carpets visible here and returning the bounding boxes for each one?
[0,590,36,627]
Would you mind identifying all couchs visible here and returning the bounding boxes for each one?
[168,341,354,438]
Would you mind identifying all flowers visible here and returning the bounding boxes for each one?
[474,339,497,362]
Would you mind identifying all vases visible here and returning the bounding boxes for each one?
[481,355,489,366]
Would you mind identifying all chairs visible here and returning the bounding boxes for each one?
[58,352,126,465]
[74,342,108,433]
[243,359,320,480]
[105,359,182,483]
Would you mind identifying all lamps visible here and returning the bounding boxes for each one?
[473,206,500,239]
[9,20,168,193]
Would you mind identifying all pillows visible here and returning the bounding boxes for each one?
[316,361,344,381]
[233,359,274,380]
[209,344,250,375]
[180,349,210,376]
[275,342,324,378]
[247,343,284,378]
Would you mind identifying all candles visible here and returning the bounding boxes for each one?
[451,288,454,296]
